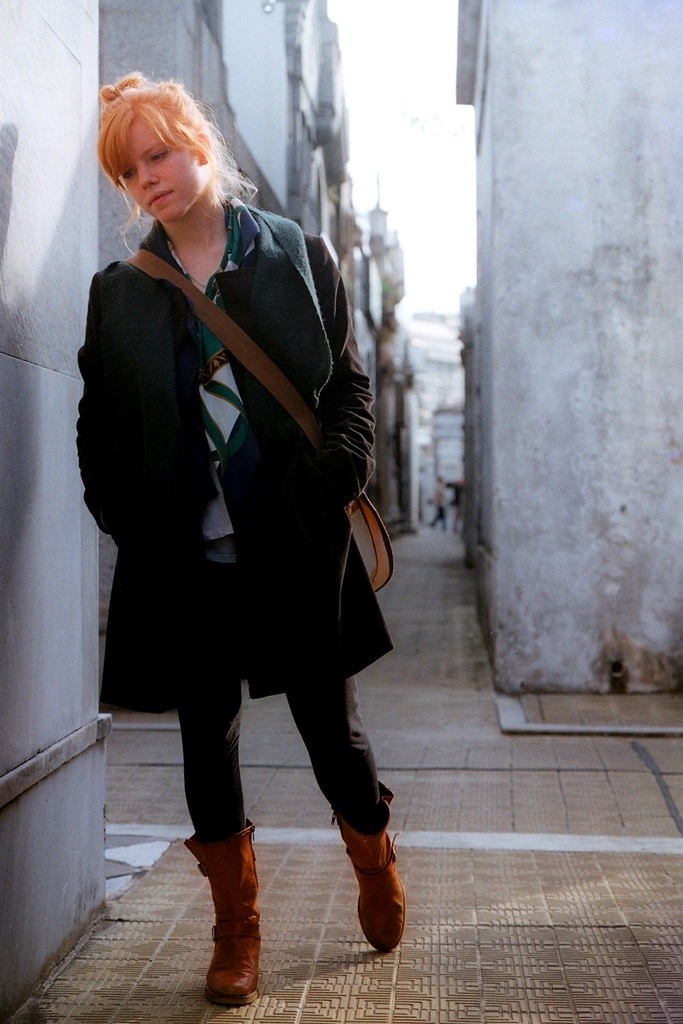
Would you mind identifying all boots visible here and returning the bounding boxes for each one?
[184,818,262,1005]
[331,778,407,953]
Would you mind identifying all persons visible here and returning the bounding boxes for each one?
[72,65,408,1005]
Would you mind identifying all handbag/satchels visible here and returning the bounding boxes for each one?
[342,491,394,592]
